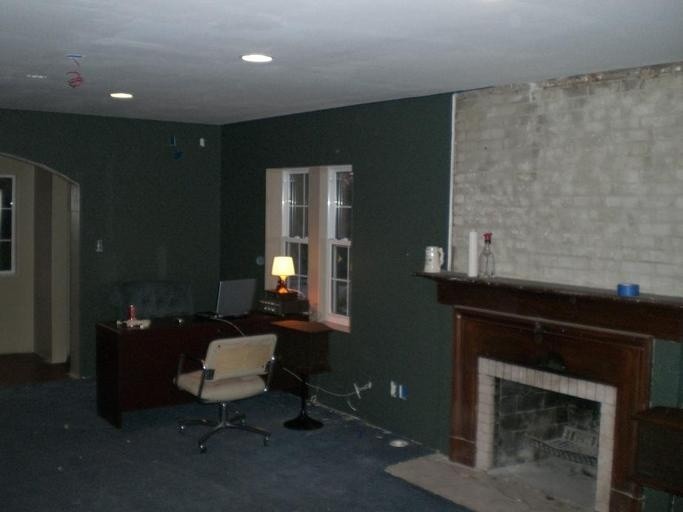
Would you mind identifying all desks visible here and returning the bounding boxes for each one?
[268,319,332,430]
[94,313,310,429]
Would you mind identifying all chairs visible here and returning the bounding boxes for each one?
[173,333,277,450]
[118,280,191,321]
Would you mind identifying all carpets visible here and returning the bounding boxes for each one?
[385,452,594,512]
[0,352,71,388]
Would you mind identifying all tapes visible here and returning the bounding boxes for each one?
[617,283,640,297]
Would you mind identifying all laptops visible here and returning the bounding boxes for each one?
[195,278,256,319]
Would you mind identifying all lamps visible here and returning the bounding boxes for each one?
[271,256,296,293]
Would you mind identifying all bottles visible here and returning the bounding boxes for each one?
[423,245,444,274]
[467,227,496,280]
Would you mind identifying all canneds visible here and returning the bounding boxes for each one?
[127,304,136,320]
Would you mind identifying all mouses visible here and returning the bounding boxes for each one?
[174,317,184,324]
